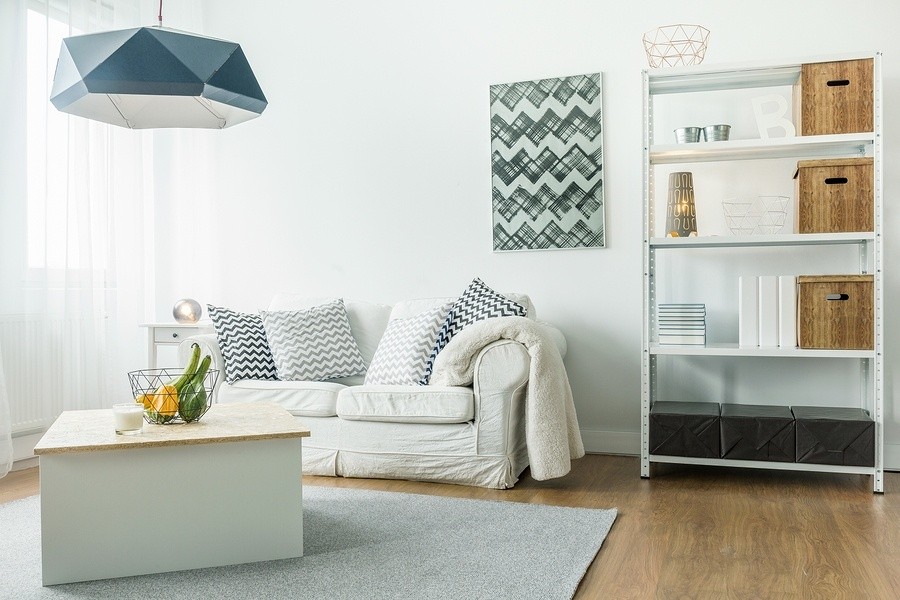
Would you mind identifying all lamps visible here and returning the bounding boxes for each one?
[50,1,268,129]
[664,171,696,238]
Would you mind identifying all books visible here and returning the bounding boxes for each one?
[738,275,797,349]
[657,303,707,346]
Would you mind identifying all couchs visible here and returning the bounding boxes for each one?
[181,310,585,489]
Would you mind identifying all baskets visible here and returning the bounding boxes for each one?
[128,368,220,425]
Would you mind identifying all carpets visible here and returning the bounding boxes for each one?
[0,486,618,600]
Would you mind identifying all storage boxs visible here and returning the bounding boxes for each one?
[793,156,873,233]
[791,57,875,135]
[652,402,875,467]
[796,273,875,349]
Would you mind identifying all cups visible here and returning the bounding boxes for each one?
[673,126,702,144]
[702,124,731,142]
[113,403,145,436]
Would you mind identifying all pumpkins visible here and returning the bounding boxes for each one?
[136,343,211,423]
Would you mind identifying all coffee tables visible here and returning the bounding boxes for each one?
[32,403,310,588]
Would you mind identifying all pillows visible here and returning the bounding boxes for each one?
[362,301,459,387]
[206,302,279,386]
[421,279,530,386]
[257,296,368,380]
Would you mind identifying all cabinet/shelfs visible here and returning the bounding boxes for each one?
[639,51,884,493]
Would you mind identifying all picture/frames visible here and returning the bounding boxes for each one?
[489,70,605,252]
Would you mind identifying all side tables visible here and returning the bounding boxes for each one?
[148,320,212,389]
